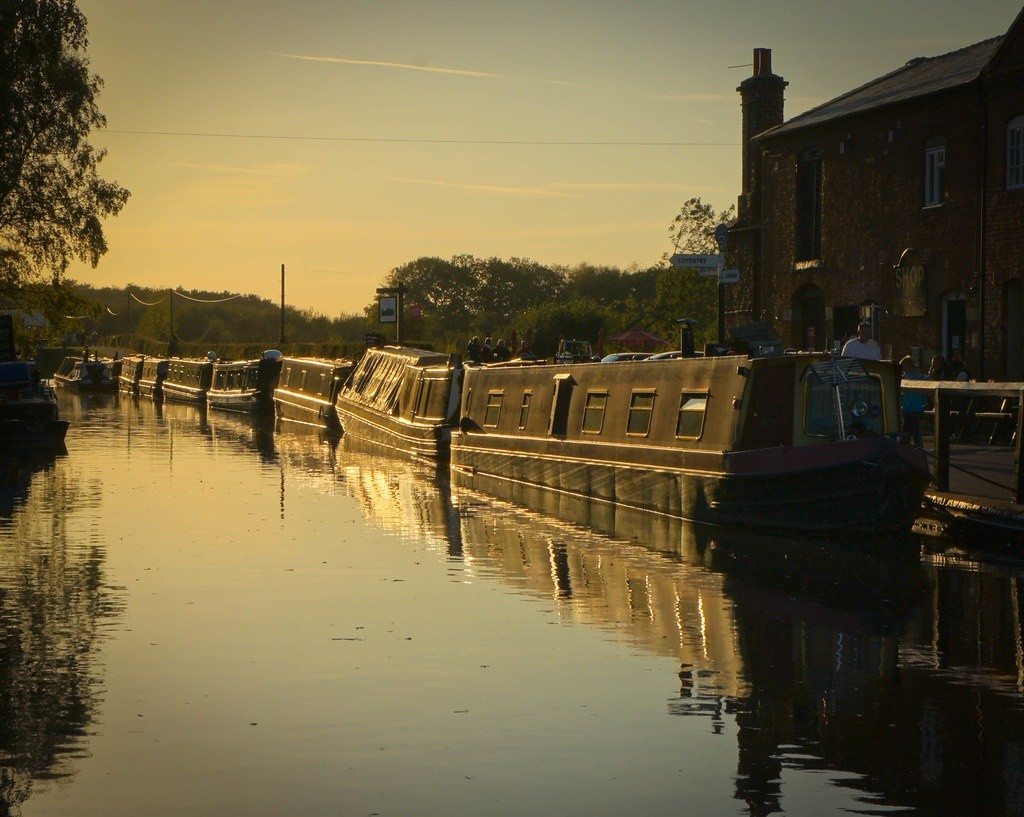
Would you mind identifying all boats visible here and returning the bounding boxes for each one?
[206,350,283,414]
[449,319,929,531]
[119,352,168,400]
[897,376,1024,538]
[336,345,465,457]
[0,313,70,453]
[272,356,358,427]
[53,345,122,392]
[161,356,214,406]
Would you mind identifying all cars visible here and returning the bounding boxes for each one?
[600,350,704,363]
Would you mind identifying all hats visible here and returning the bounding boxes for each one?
[473,336,478,342]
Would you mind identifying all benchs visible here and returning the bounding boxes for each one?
[925,399,1013,444]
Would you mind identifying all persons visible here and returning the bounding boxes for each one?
[467,336,482,361]
[517,340,532,357]
[926,355,961,409]
[480,337,492,363]
[490,339,510,363]
[899,355,925,450]
[951,360,970,382]
[841,322,882,360]
